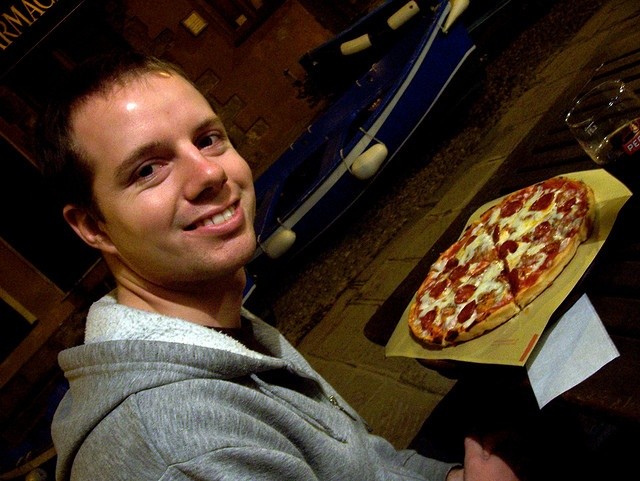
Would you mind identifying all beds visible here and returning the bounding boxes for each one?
[363,20,638,421]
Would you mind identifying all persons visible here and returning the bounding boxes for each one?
[31,35,540,481]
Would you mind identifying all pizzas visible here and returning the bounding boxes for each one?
[408,177,595,348]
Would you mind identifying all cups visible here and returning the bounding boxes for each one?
[564,79,640,191]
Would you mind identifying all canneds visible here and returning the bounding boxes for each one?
[565,80,640,165]
